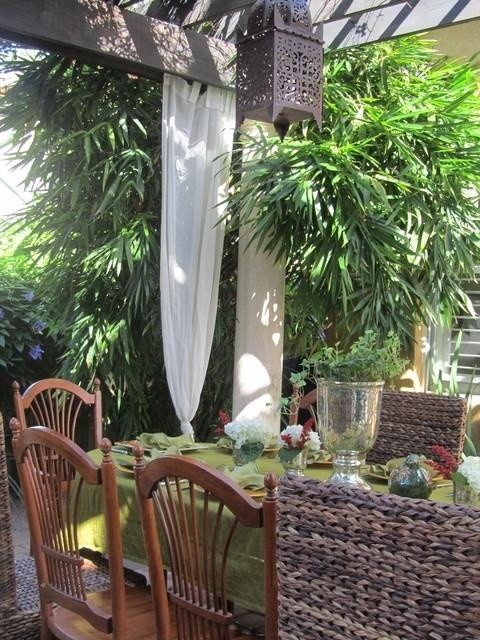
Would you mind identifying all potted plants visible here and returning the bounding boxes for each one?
[290,328,410,492]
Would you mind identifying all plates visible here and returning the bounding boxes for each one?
[114,436,444,499]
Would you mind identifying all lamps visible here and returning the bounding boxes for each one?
[235,1,326,143]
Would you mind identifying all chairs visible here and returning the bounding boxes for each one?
[11,378,105,558]
[130,439,277,638]
[7,414,217,639]
[273,473,480,639]
[364,389,470,469]
